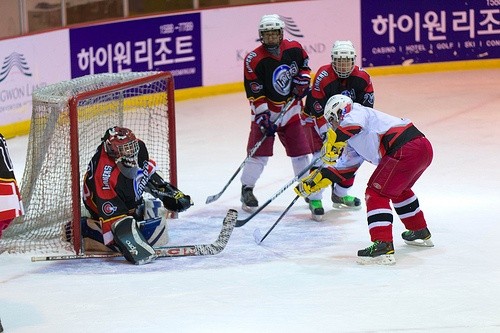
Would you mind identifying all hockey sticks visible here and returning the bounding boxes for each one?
[233,154,325,228]
[31,209,239,262]
[206,94,296,203]
[252,162,325,245]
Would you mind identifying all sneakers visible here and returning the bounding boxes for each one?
[401,226,435,247]
[355,239,397,265]
[306,198,325,222]
[329,184,362,210]
[240,185,260,213]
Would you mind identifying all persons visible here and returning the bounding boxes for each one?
[0,134,25,239]
[240,14,312,211]
[294,95,434,265]
[300,40,375,221]
[61,126,194,266]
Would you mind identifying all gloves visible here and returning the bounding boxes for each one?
[292,168,332,200]
[321,128,347,167]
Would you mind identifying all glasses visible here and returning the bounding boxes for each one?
[263,31,280,40]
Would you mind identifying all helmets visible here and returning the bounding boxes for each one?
[331,39,357,79]
[322,94,353,128]
[258,14,286,49]
[103,125,139,170]
